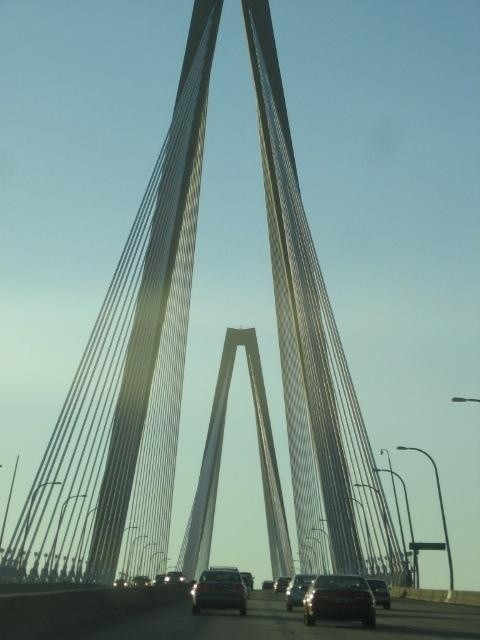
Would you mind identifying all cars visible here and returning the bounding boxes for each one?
[191,567,247,615]
[274,577,291,592]
[185,581,196,600]
[240,571,253,591]
[113,576,151,589]
[365,577,391,609]
[302,575,377,628]
[165,571,186,587]
[153,573,166,584]
[263,581,273,590]
[286,574,317,611]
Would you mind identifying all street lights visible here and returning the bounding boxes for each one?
[354,483,401,584]
[51,494,88,586]
[372,467,419,589]
[343,496,377,579]
[20,480,61,585]
[73,505,108,586]
[115,527,170,575]
[291,507,350,575]
[396,446,456,604]
